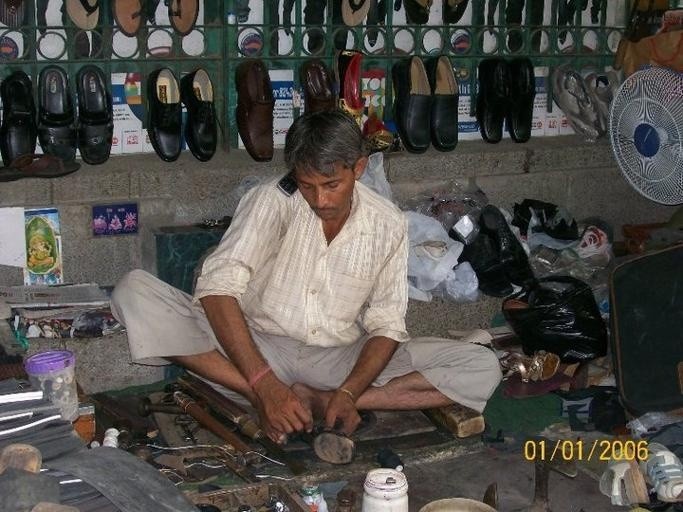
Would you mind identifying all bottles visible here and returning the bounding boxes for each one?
[329,488,359,512]
[296,485,329,512]
[360,465,416,510]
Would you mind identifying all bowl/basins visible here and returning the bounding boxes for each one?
[417,497,497,512]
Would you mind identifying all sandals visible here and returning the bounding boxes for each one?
[496,350,561,385]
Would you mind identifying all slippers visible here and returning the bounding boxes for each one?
[343,2,371,26]
[62,2,100,31]
[0,153,79,181]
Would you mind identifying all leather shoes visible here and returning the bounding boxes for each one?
[146,68,219,163]
[236,60,276,162]
[0,65,110,165]
[474,205,538,288]
[448,211,513,298]
[390,54,461,156]
[301,56,336,115]
[476,57,536,145]
[71,313,121,339]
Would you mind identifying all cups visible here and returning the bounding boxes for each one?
[23,347,78,425]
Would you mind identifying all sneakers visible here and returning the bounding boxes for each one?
[637,440,683,506]
[598,449,649,512]
[549,65,623,142]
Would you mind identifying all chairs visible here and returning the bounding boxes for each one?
[609,244,683,453]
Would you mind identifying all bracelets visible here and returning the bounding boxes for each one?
[337,385,358,404]
[247,368,270,387]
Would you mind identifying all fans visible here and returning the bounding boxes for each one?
[609,66,682,206]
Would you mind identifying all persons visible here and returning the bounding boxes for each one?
[109,107,501,441]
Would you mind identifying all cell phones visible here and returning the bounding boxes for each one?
[278,171,299,197]
